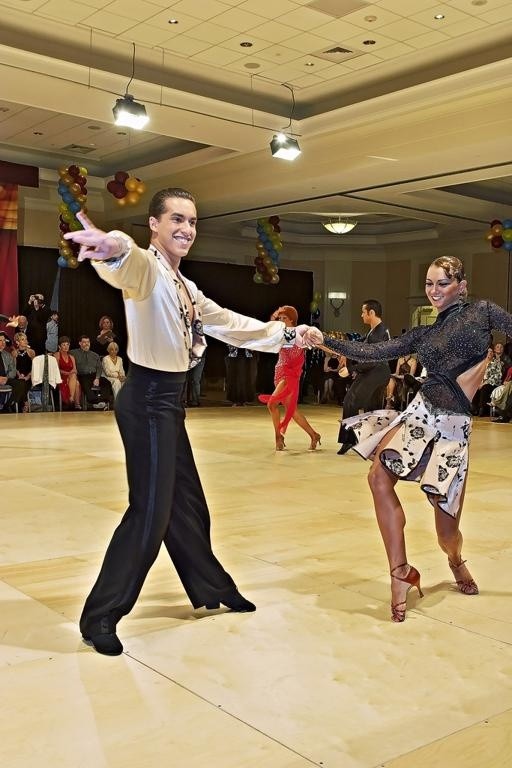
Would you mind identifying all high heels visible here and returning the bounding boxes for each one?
[390,563,423,623]
[308,433,321,450]
[82,632,122,654]
[448,554,478,594]
[276,435,286,450]
[206,589,256,612]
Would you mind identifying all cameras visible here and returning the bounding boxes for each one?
[4,338,12,346]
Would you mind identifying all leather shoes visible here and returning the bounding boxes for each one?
[338,443,354,454]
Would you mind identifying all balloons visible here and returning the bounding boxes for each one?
[251,215,281,286]
[485,219,512,253]
[106,172,147,209]
[56,164,89,270]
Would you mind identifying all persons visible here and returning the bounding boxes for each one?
[337,297,393,457]
[184,334,206,408]
[301,253,511,625]
[224,343,254,405]
[471,339,512,422]
[303,331,363,408]
[64,186,325,655]
[0,293,126,414]
[385,353,427,414]
[256,304,324,452]
